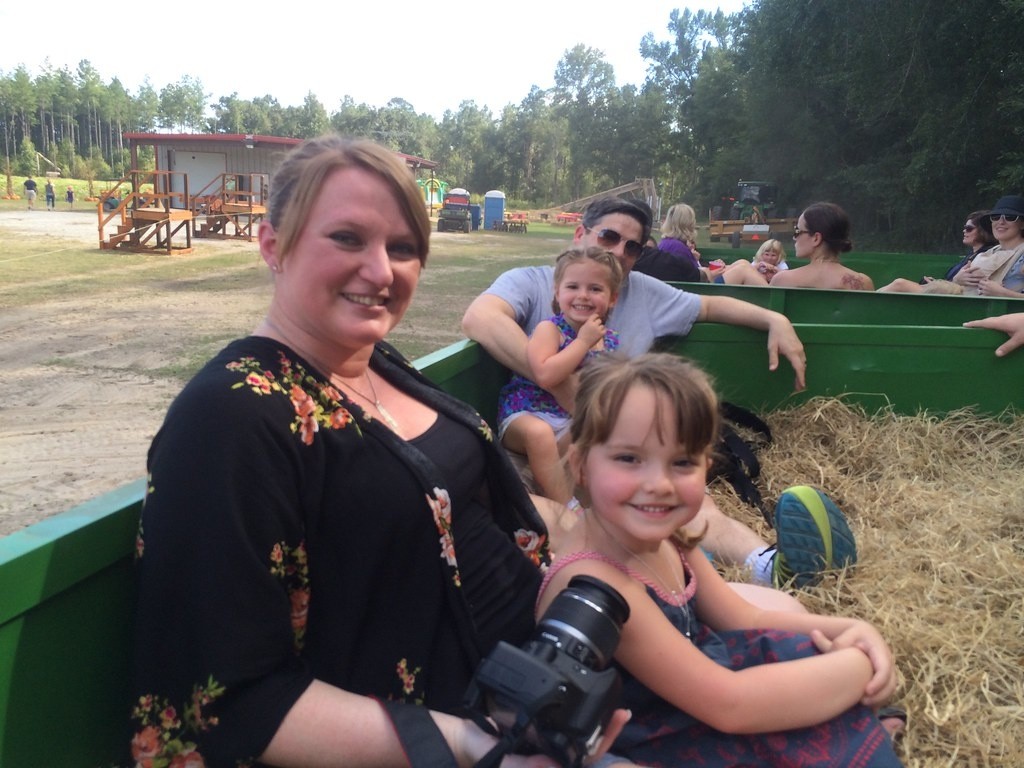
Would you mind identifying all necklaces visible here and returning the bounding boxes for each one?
[591,508,706,641]
[265,316,397,429]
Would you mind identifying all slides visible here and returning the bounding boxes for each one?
[552,181,640,211]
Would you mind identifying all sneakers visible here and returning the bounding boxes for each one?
[773,484,858,596]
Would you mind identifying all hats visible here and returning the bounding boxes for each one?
[984,195,1024,216]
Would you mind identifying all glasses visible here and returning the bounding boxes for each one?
[793,225,824,238]
[960,224,977,232]
[585,225,645,260]
[990,214,1020,223]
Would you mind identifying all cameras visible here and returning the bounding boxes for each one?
[472,574,630,768]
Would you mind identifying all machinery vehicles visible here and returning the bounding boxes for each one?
[707,179,800,243]
[436,193,473,234]
[731,206,779,249]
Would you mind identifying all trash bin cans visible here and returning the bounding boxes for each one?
[469,203,482,231]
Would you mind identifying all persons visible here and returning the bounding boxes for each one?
[535,354,906,768]
[24,175,38,210]
[460,197,857,599]
[45,179,56,211]
[750,239,789,285]
[769,203,874,291]
[629,199,769,285]
[963,313,1024,357]
[875,196,1024,298]
[66,185,74,208]
[498,246,624,516]
[116,133,641,768]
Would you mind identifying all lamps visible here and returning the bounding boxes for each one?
[244,139,254,149]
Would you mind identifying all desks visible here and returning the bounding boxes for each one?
[557,213,583,223]
[493,219,528,234]
